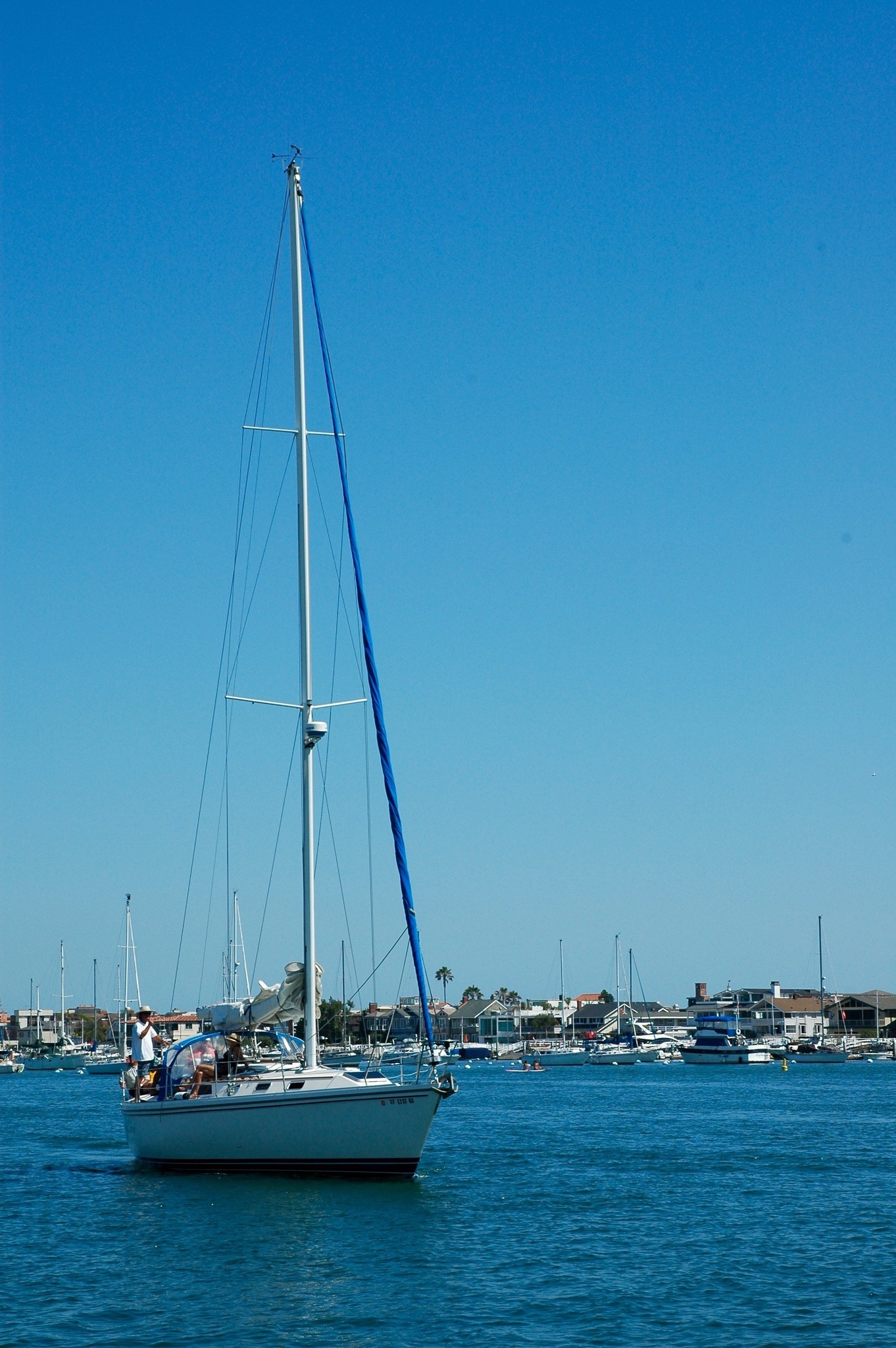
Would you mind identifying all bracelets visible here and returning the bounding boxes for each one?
[161,1040,163,1042]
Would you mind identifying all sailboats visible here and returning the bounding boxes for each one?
[1,891,461,1075]
[118,149,458,1186]
[522,916,851,1066]
[860,992,893,1060]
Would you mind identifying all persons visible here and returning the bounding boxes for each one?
[180,1033,249,1099]
[533,1060,541,1070]
[125,1055,151,1097]
[188,1037,216,1062]
[131,1005,171,1102]
[523,1061,529,1071]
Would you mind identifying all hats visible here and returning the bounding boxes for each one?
[135,1006,155,1017]
[128,1050,132,1057]
[224,1033,241,1044]
[205,1038,210,1041]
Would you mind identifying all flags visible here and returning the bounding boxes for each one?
[843,1011,846,1019]
[830,1011,834,1019]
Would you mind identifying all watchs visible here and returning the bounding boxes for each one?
[140,1084,143,1088]
